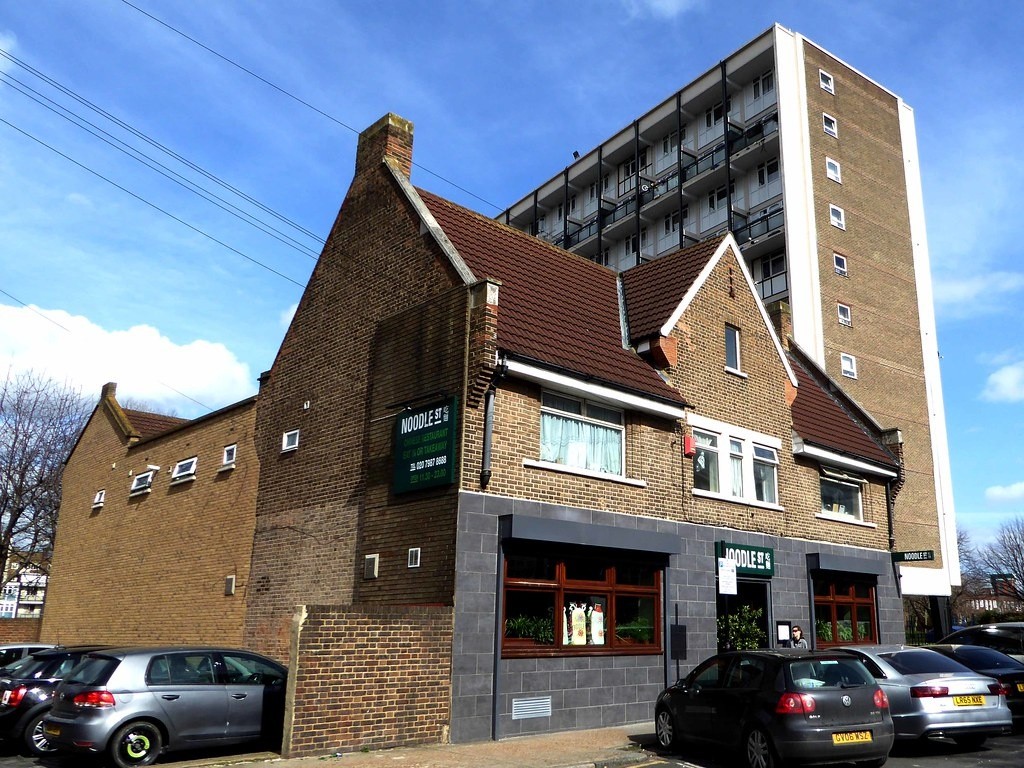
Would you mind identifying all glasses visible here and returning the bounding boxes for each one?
[792,629,800,632]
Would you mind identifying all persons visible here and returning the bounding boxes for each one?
[786,625,808,649]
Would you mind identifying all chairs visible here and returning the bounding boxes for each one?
[170,656,200,685]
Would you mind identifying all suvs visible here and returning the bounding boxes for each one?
[654,648,895,768]
[41,646,290,768]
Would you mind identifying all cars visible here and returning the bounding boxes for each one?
[0,641,129,758]
[916,645,1024,735]
[829,645,1012,753]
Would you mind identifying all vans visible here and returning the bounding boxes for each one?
[934,622,1024,666]
